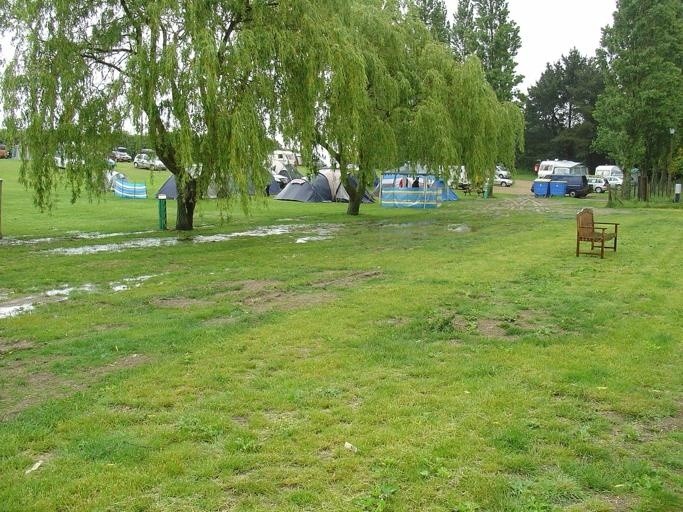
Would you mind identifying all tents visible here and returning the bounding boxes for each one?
[102,170,126,190]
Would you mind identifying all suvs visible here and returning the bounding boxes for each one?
[106,146,166,170]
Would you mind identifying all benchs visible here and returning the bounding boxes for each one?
[574,207,619,258]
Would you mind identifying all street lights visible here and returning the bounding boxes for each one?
[669,126,675,195]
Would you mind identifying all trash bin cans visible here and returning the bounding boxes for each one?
[533,178,568,198]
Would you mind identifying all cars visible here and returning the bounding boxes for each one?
[531,175,637,198]
[273,151,297,167]
[448,166,513,190]
